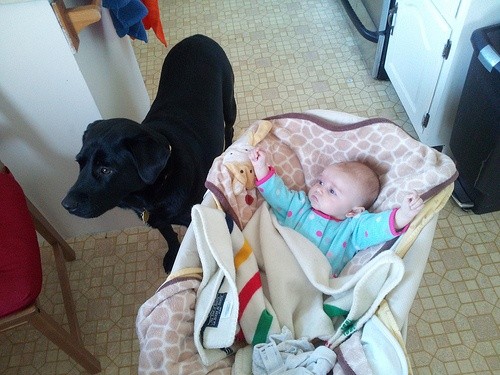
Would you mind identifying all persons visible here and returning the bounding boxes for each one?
[249,147,423,348]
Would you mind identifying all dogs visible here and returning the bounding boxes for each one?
[59,33,237,277]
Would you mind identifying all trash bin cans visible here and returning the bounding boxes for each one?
[439,23,500,215]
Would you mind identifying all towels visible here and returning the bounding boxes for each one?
[189,199,412,375]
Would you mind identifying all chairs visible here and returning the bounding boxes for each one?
[0,161,102,374]
[137,109,460,375]
